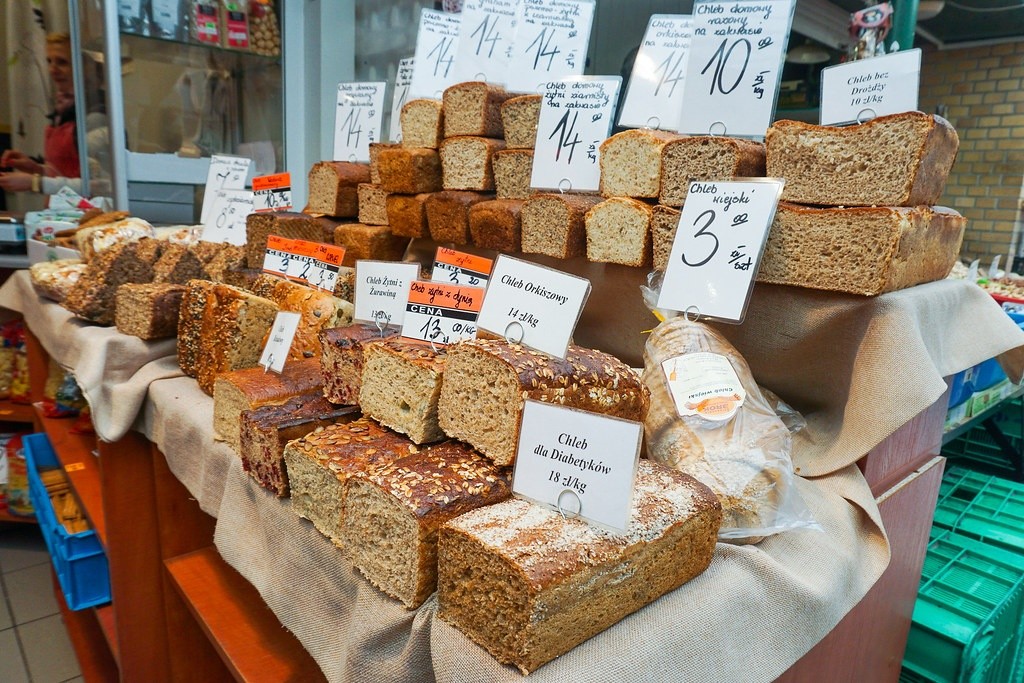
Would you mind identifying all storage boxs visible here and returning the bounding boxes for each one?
[897,308,1024,683]
[23,432,114,613]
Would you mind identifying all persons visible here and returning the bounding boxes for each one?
[0,31,123,209]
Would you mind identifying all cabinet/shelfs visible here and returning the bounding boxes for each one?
[0,269,431,683]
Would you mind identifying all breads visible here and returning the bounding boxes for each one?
[302,80,969,297]
[28,211,791,676]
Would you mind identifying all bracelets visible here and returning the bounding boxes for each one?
[32,173,41,193]
